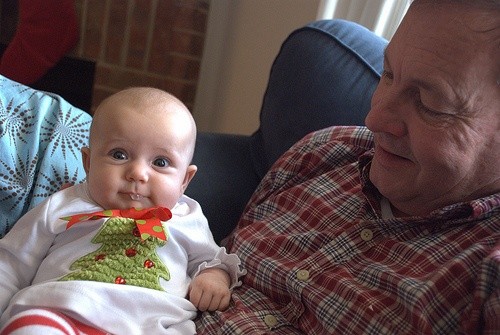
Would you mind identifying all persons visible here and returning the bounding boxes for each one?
[0,85,248,335]
[194,0,500,335]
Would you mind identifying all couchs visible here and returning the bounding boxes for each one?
[183,19,391,249]
